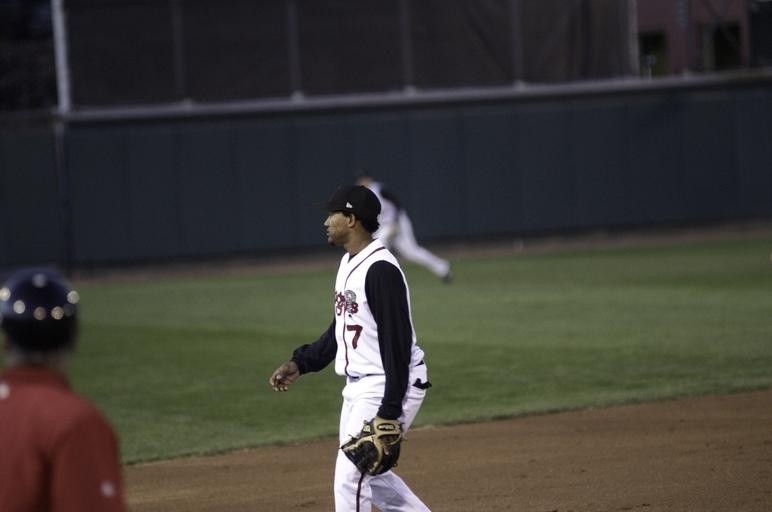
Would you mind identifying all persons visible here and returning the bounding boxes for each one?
[0,263,125,510]
[353,177,453,284]
[271,184,434,510]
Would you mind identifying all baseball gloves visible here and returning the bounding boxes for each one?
[341,419,404,476]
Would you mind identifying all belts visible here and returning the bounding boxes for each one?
[349,357,424,379]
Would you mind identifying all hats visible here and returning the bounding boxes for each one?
[308,182,382,227]
[0,265,79,354]
